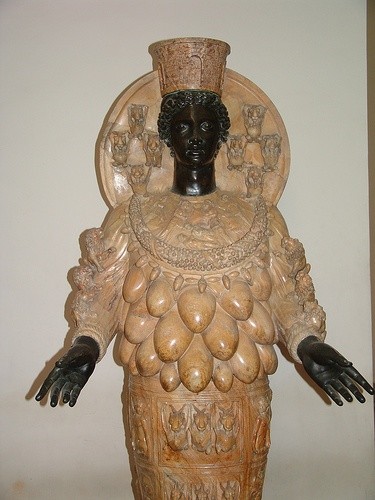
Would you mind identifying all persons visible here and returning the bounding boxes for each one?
[28,92,375,500]
